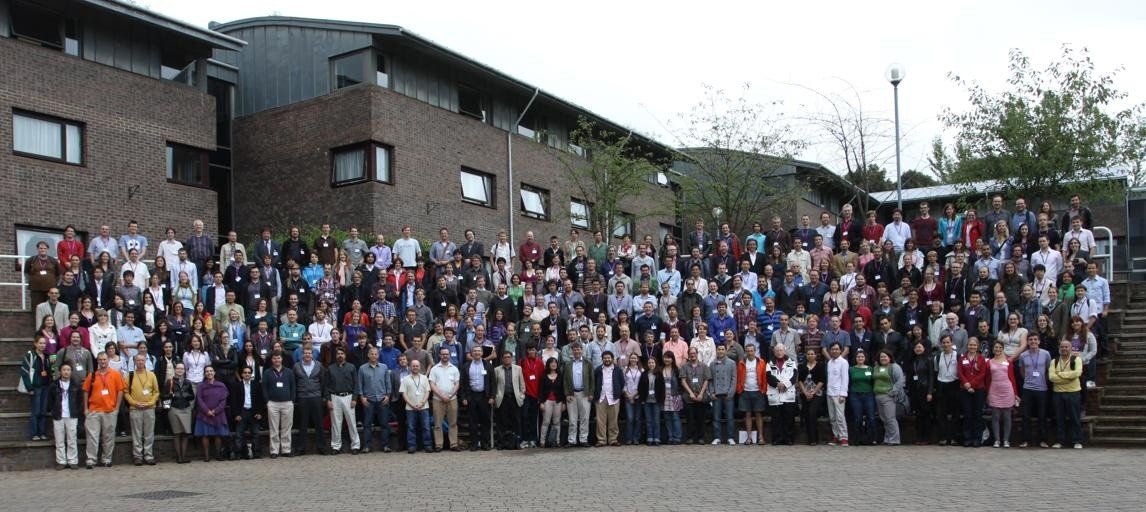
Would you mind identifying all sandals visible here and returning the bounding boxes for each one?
[758,436,765,444]
[744,438,753,445]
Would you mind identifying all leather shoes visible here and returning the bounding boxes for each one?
[498,443,507,450]
[436,447,442,452]
[254,453,264,459]
[331,449,340,455]
[352,449,359,454]
[236,453,246,460]
[183,458,191,463]
[541,442,547,448]
[408,447,417,454]
[294,450,305,456]
[283,452,292,457]
[482,442,493,450]
[595,442,603,447]
[612,441,621,446]
[452,445,462,451]
[319,447,329,455]
[426,447,434,452]
[176,457,183,463]
[271,453,279,458]
[216,456,225,461]
[554,443,562,448]
[384,445,393,453]
[362,447,370,453]
[470,444,479,451]
[204,456,210,461]
[973,440,983,446]
[513,441,521,449]
[582,441,592,447]
[964,439,972,445]
[564,442,574,448]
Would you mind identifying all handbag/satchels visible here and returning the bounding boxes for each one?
[17,350,34,394]
[163,380,173,410]
[123,370,134,414]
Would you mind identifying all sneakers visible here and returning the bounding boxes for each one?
[1003,440,1010,448]
[1020,441,1032,448]
[840,438,849,446]
[655,441,661,446]
[135,459,143,466]
[672,440,681,445]
[727,439,736,445]
[56,464,67,470]
[88,463,95,469]
[712,438,722,445]
[994,440,1001,448]
[938,439,949,445]
[102,461,112,467]
[687,438,694,444]
[665,440,674,444]
[1039,442,1049,447]
[646,441,652,445]
[520,440,529,449]
[32,435,40,441]
[698,438,706,444]
[530,440,537,448]
[145,459,157,464]
[949,438,962,445]
[41,435,49,440]
[1075,443,1084,449]
[69,463,79,469]
[827,437,839,446]
[634,439,641,445]
[1051,443,1063,449]
[626,440,633,445]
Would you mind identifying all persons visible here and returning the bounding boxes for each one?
[19,195,1111,468]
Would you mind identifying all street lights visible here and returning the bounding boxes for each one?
[885,61,904,210]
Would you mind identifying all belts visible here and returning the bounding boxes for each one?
[575,390,584,392]
[332,393,352,397]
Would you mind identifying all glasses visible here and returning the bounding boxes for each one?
[952,266,963,269]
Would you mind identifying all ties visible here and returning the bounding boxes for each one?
[265,240,269,252]
